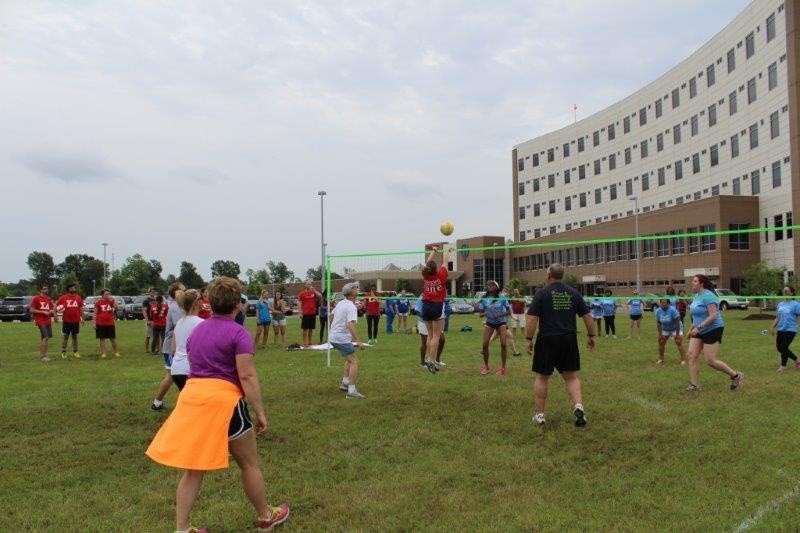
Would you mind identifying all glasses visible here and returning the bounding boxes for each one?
[372,289,376,291]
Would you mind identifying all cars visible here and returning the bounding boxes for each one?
[628,294,663,312]
[81,294,146,321]
[246,294,299,316]
[448,298,475,314]
[714,288,749,311]
[331,289,419,316]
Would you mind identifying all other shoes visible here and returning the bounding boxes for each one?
[61,352,67,359]
[367,339,378,345]
[434,360,447,367]
[419,364,427,367]
[112,351,120,357]
[40,355,51,362]
[573,405,586,426]
[776,365,787,372]
[531,412,546,424]
[480,367,490,375]
[425,361,436,374]
[495,368,506,376]
[794,360,800,370]
[150,403,171,413]
[100,352,107,358]
[73,350,80,358]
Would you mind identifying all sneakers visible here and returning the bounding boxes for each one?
[685,383,700,392]
[340,382,357,390]
[174,523,208,533]
[344,392,366,399]
[730,370,744,390]
[257,502,289,533]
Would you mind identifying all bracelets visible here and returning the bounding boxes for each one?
[587,334,596,337]
[526,337,532,340]
[697,324,703,331]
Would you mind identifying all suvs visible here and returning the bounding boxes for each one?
[0,296,34,322]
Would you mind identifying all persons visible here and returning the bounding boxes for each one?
[93,288,121,358]
[685,273,745,393]
[477,279,512,377]
[772,285,800,374]
[28,285,53,362]
[142,243,689,425]
[145,276,289,533]
[55,284,86,359]
[327,280,365,399]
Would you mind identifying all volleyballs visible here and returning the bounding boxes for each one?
[440,221,455,236]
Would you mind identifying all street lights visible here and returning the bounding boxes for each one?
[628,194,641,295]
[53,285,57,294]
[317,190,327,294]
[493,242,499,284]
[100,242,108,291]
[92,280,96,296]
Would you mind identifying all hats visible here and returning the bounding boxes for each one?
[487,279,499,288]
[342,281,360,296]
